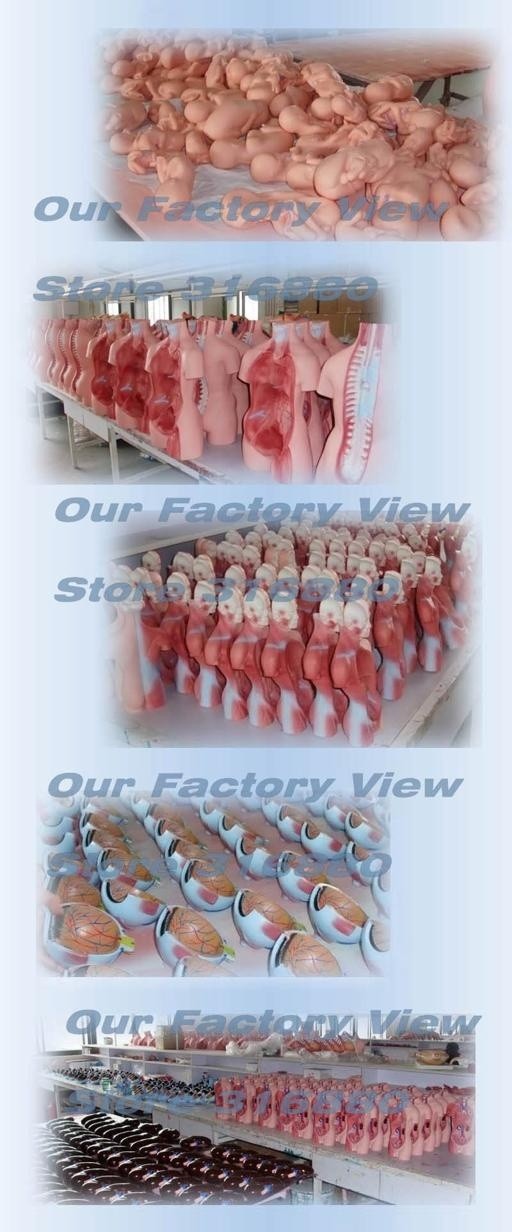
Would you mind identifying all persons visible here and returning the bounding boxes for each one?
[95,523,477,748]
[215,1073,476,1162]
[25,308,394,482]
[99,31,505,240]
